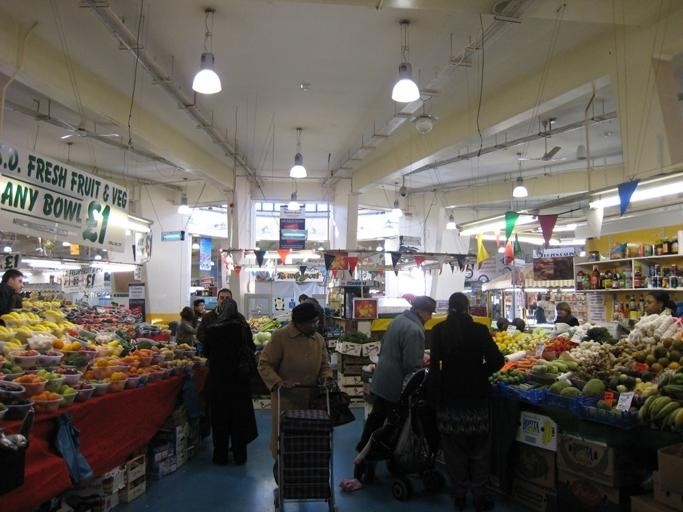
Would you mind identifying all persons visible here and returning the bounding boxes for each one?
[357,297,436,471]
[192,298,208,331]
[176,306,202,351]
[198,289,256,353]
[202,298,254,466]
[0,269,25,327]
[298,294,326,336]
[641,290,677,317]
[430,293,504,511]
[556,302,580,326]
[257,303,329,483]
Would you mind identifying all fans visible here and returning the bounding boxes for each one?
[519,121,567,162]
[55,117,121,140]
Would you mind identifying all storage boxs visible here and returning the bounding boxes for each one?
[653,472,683,510]
[125,453,146,482]
[557,433,629,486]
[337,353,377,375]
[659,442,682,491]
[630,494,683,512]
[64,492,119,512]
[515,410,559,451]
[146,410,206,480]
[337,339,361,357]
[362,340,381,356]
[511,445,558,496]
[510,480,554,512]
[556,470,629,512]
[120,476,146,504]
[337,372,366,408]
[66,469,125,495]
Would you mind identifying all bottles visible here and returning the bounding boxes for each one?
[608,292,647,328]
[583,233,681,263]
[632,255,683,291]
[575,260,633,291]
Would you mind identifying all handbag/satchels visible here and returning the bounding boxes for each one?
[236,327,257,378]
[308,377,355,427]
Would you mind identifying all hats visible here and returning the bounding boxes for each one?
[411,296,436,313]
[292,303,320,322]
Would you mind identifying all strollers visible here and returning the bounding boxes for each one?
[371,368,446,501]
[274,381,335,507]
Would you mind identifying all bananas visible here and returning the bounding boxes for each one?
[0,325,16,338]
[44,309,64,320]
[2,312,25,325]
[3,342,24,354]
[34,324,53,336]
[17,326,36,339]
[44,320,60,332]
[24,311,42,325]
[637,397,683,435]
[61,320,77,329]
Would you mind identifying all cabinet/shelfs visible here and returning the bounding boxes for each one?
[574,225,683,325]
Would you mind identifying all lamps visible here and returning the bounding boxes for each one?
[410,98,437,134]
[446,208,455,230]
[176,181,190,216]
[392,19,420,103]
[288,180,300,212]
[391,183,402,219]
[513,152,529,198]
[290,127,309,178]
[191,8,223,95]
[587,179,683,209]
[457,208,542,237]
